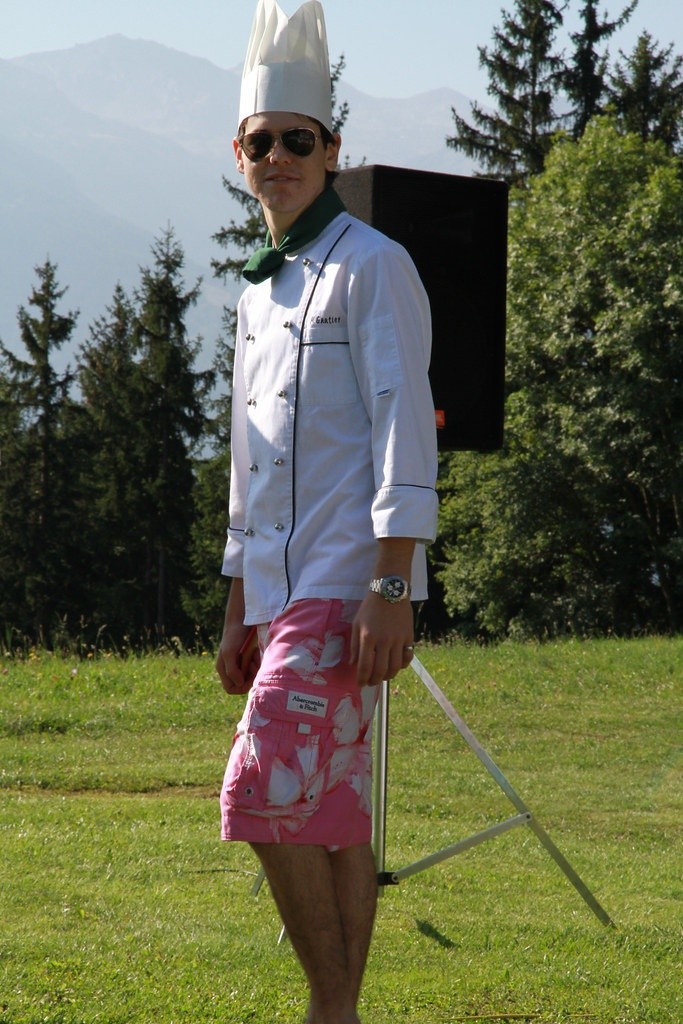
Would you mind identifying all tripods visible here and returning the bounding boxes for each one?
[251,653,622,937]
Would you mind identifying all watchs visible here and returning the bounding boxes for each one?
[366,576,413,604]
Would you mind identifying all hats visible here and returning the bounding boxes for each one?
[238,0,332,134]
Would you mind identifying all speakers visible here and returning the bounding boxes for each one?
[330,165,510,453]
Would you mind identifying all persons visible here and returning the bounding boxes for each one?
[213,62,442,1024]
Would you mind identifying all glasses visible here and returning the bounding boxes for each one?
[239,127,321,163]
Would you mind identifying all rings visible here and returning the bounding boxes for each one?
[404,645,415,651]
